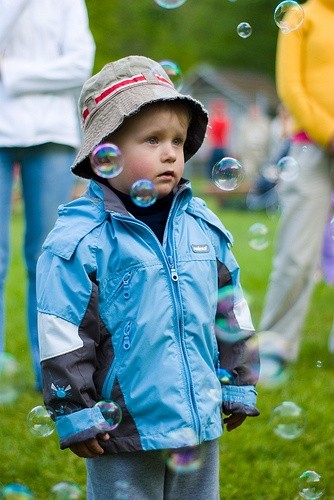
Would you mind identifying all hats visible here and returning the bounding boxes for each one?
[71,55,210,179]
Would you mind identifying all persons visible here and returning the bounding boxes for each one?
[260,0,333,379]
[35,56,262,499]
[201,94,290,188]
[0,0,96,393]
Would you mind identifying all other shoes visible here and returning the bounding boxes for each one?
[257,356,290,391]
[0,352,16,375]
